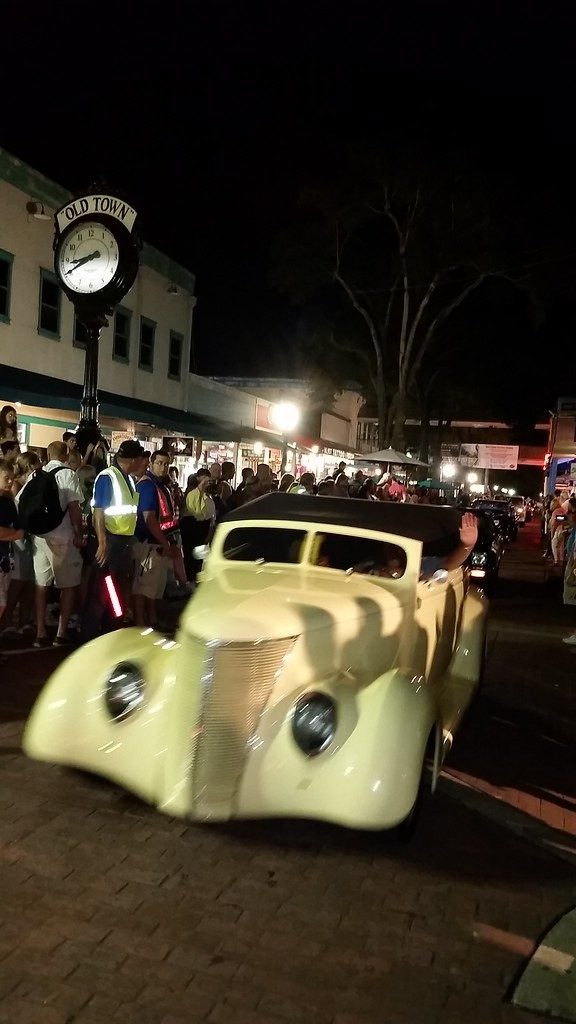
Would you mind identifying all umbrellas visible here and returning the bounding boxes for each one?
[349,446,431,473]
[418,477,455,490]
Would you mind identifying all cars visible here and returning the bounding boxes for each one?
[462,508,503,597]
[471,499,518,545]
[20,492,488,844]
[493,495,526,528]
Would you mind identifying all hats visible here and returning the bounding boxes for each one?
[119,440,144,458]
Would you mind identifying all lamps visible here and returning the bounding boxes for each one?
[26,200,51,220]
[58,222,119,294]
[167,283,179,296]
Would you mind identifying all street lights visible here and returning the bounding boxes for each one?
[276,404,298,476]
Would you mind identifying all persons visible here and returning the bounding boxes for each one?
[543,481,576,645]
[0,405,489,648]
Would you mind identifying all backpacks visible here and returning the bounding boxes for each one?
[18,466,75,535]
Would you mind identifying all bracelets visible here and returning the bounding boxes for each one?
[462,545,473,551]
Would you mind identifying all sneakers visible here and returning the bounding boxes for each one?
[53,637,73,646]
[562,634,576,645]
[34,636,52,647]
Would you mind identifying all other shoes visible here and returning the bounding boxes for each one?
[155,627,174,636]
